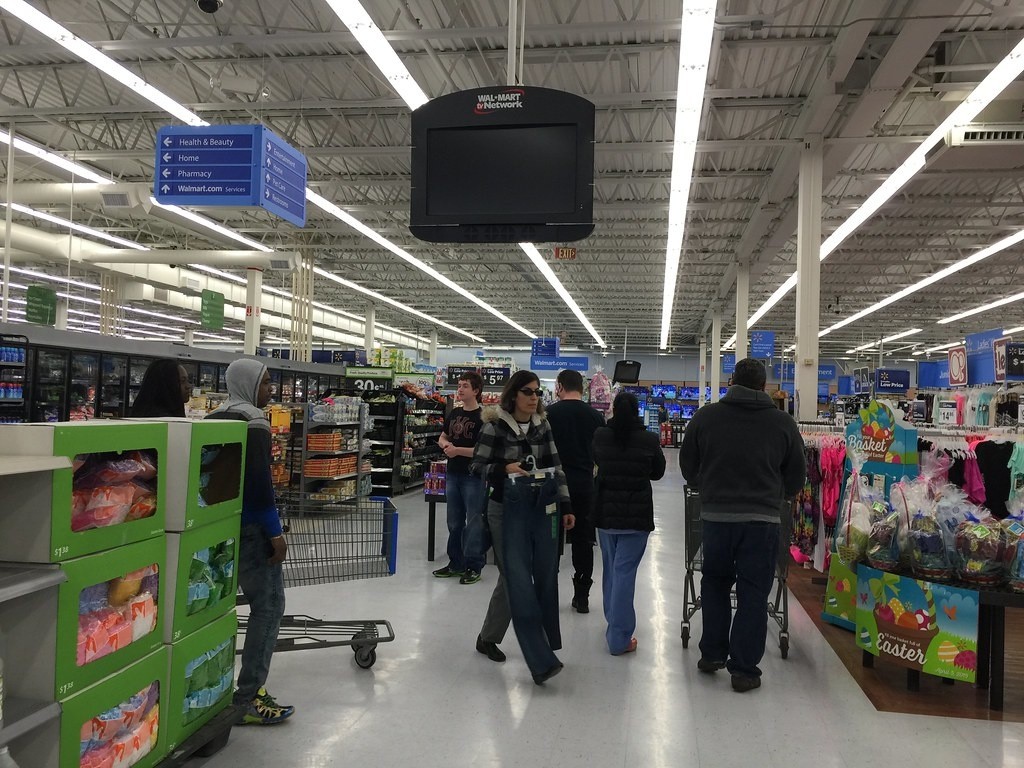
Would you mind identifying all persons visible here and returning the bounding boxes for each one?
[127,360,192,418]
[592,392,666,655]
[432,371,487,584]
[680,358,806,691]
[468,370,576,662]
[544,370,606,613]
[198,358,295,725]
[658,406,667,424]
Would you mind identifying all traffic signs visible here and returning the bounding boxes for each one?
[261,185,306,228]
[154,166,261,184]
[262,169,307,207]
[154,182,260,206]
[262,125,309,179]
[258,151,307,192]
[532,337,556,356]
[155,123,262,149]
[155,149,261,167]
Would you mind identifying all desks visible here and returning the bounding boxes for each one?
[424,495,448,561]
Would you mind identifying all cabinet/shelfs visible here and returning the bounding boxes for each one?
[274,402,365,519]
[0,454,62,744]
[325,388,453,498]
[188,401,296,528]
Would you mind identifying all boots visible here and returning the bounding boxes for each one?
[572,577,593,612]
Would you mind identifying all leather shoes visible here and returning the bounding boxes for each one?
[476,633,506,662]
[535,663,563,684]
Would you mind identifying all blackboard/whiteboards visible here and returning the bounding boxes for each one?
[409,85,595,242]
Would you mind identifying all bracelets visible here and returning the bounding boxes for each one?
[270,535,282,540]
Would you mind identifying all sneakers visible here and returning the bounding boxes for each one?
[232,688,294,725]
[460,566,480,584]
[433,565,466,578]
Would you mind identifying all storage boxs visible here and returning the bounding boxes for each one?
[0,417,249,768]
[423,461,446,496]
[304,432,357,477]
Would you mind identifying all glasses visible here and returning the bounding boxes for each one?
[518,389,543,397]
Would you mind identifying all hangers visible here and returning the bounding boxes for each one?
[799,424,848,449]
[912,422,1024,461]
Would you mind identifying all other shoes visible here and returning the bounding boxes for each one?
[731,673,761,691]
[612,637,637,656]
[698,656,726,670]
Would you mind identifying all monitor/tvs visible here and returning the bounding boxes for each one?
[612,360,642,384]
[407,86,595,243]
[704,387,728,402]
[625,385,700,418]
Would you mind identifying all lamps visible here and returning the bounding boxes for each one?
[0,0,607,350]
[661,0,1024,355]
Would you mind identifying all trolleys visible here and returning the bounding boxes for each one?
[236,490,401,670]
[682,483,793,660]
